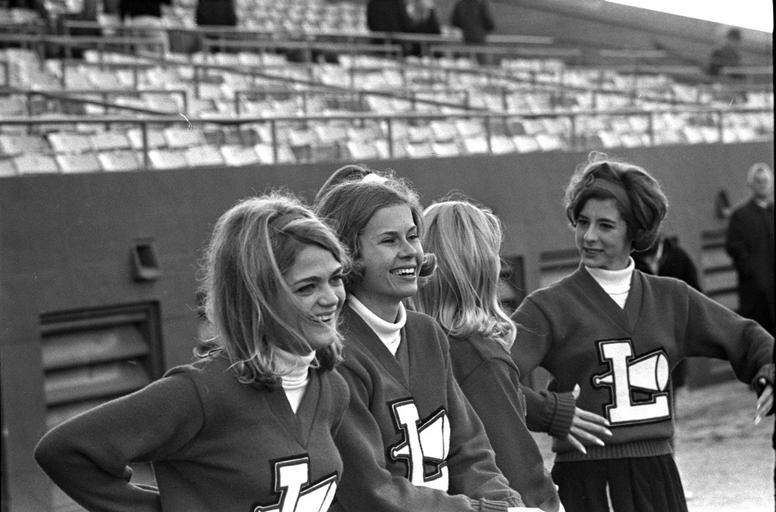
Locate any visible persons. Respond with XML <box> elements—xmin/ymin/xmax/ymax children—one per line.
<box><xmin>308</xmin><ymin>164</ymin><xmax>371</xmax><ymax>213</ymax></box>
<box><xmin>312</xmin><ymin>169</ymin><xmax>542</xmax><ymax>511</ymax></box>
<box><xmin>368</xmin><ymin>0</ymin><xmax>497</xmax><ymax>66</ymax></box>
<box><xmin>701</xmin><ymin>25</ymin><xmax>749</xmax><ymax>102</ymax></box>
<box><xmin>640</xmin><ymin>236</ymin><xmax>706</xmax><ymax>392</ymax></box>
<box><xmin>510</xmin><ymin>151</ymin><xmax>776</xmax><ymax>512</ymax></box>
<box><xmin>724</xmin><ymin>161</ymin><xmax>775</xmax><ymax>338</ymax></box>
<box><xmin>713</xmin><ymin>187</ymin><xmax>732</xmax><ymax>220</ymax></box>
<box><xmin>33</xmin><ymin>193</ymin><xmax>351</xmax><ymax>512</ymax></box>
<box><xmin>402</xmin><ymin>199</ymin><xmax>564</xmax><ymax>512</ymax></box>
<box><xmin>0</xmin><ymin>0</ymin><xmax>238</xmax><ymax>60</ymax></box>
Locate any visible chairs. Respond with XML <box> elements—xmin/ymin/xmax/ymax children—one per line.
<box><xmin>0</xmin><ymin>0</ymin><xmax>775</xmax><ymax>181</ymax></box>
